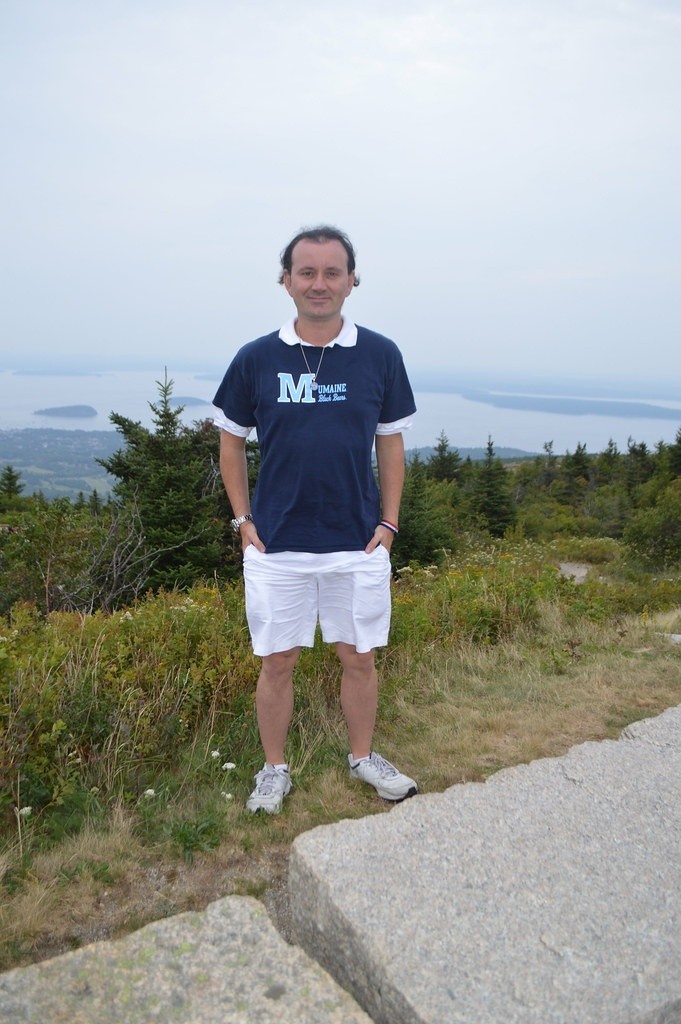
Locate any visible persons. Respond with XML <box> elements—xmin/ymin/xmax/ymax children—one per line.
<box><xmin>212</xmin><ymin>228</ymin><xmax>417</xmax><ymax>815</ymax></box>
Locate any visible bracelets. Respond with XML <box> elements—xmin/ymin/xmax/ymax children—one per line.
<box><xmin>380</xmin><ymin>520</ymin><xmax>398</xmax><ymax>534</ymax></box>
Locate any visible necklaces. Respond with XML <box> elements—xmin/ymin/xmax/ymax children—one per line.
<box><xmin>298</xmin><ymin>332</ymin><xmax>326</xmax><ymax>391</ymax></box>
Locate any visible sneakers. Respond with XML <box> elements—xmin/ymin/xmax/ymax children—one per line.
<box><xmin>348</xmin><ymin>752</ymin><xmax>418</xmax><ymax>801</ymax></box>
<box><xmin>246</xmin><ymin>762</ymin><xmax>293</xmax><ymax>816</ymax></box>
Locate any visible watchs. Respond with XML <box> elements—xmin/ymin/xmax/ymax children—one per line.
<box><xmin>231</xmin><ymin>514</ymin><xmax>252</xmax><ymax>533</ymax></box>
